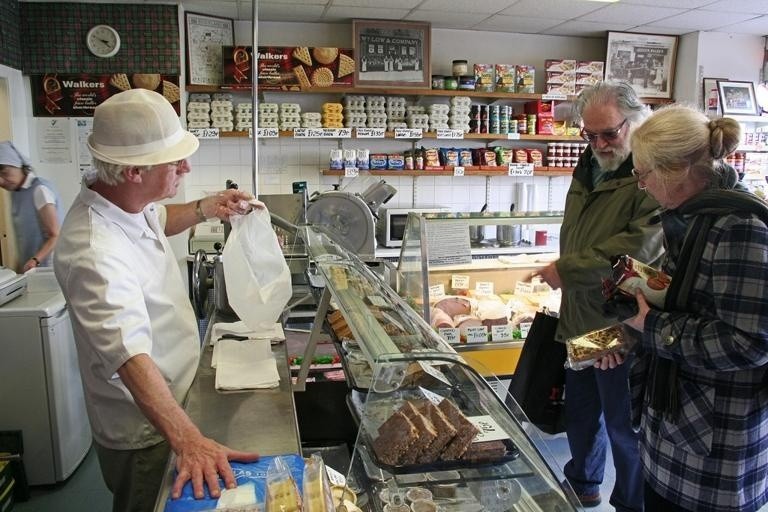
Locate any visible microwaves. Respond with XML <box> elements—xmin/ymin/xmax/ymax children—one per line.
<box><xmin>374</xmin><ymin>206</ymin><xmax>461</xmax><ymax>249</ymax></box>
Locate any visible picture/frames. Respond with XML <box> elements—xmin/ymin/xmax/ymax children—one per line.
<box><xmin>702</xmin><ymin>77</ymin><xmax>728</xmax><ymax>118</ymax></box>
<box><xmin>352</xmin><ymin>17</ymin><xmax>432</xmax><ymax>91</ymax></box>
<box><xmin>182</xmin><ymin>10</ymin><xmax>236</xmax><ymax>88</ymax></box>
<box><xmin>185</xmin><ymin>81</ymin><xmax>674</xmax><ymax>179</ymax></box>
<box><xmin>715</xmin><ymin>79</ymin><xmax>757</xmax><ymax>116</ymax></box>
<box><xmin>603</xmin><ymin>31</ymin><xmax>679</xmax><ymax>100</ymax></box>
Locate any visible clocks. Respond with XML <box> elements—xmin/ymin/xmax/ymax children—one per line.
<box><xmin>87</xmin><ymin>25</ymin><xmax>122</xmax><ymax>59</ymax></box>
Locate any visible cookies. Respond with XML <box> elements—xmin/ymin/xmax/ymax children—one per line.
<box><xmin>133</xmin><ymin>74</ymin><xmax>160</xmax><ymax>91</ymax></box>
<box><xmin>294</xmin><ymin>65</ymin><xmax>311</xmax><ymax>88</ymax></box>
<box><xmin>292</xmin><ymin>47</ymin><xmax>312</xmax><ymax>66</ymax></box>
<box><xmin>314</xmin><ymin>48</ymin><xmax>338</xmax><ymax>64</ymax></box>
<box><xmin>338</xmin><ymin>54</ymin><xmax>355</xmax><ymax>78</ymax></box>
<box><xmin>313</xmin><ymin>68</ymin><xmax>333</xmax><ymax>88</ymax></box>
<box><xmin>109</xmin><ymin>73</ymin><xmax>131</xmax><ymax>91</ymax></box>
<box><xmin>163</xmin><ymin>81</ymin><xmax>180</xmax><ymax>103</ymax></box>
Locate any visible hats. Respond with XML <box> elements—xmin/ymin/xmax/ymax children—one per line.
<box><xmin>87</xmin><ymin>88</ymin><xmax>199</xmax><ymax>165</ymax></box>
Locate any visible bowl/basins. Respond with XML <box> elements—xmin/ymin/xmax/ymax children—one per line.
<box><xmin>378</xmin><ymin>487</ymin><xmax>438</xmax><ymax>512</ymax></box>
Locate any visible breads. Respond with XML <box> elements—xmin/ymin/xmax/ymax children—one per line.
<box><xmin>329</xmin><ymin>308</ymin><xmax>352</xmax><ymax>339</ymax></box>
<box><xmin>373</xmin><ymin>398</ymin><xmax>507</xmax><ymax>465</ymax></box>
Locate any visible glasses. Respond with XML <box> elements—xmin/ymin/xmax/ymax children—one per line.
<box><xmin>580</xmin><ymin>119</ymin><xmax>626</xmax><ymax>141</ymax></box>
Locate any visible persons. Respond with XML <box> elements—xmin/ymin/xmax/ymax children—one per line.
<box><xmin>590</xmin><ymin>102</ymin><xmax>768</xmax><ymax>511</ymax></box>
<box><xmin>523</xmin><ymin>80</ymin><xmax>666</xmax><ymax>512</ymax></box>
<box><xmin>52</xmin><ymin>88</ymin><xmax>268</xmax><ymax>510</ymax></box>
<box><xmin>0</xmin><ymin>139</ymin><xmax>69</xmax><ymax>275</ymax></box>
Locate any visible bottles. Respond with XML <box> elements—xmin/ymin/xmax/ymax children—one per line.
<box><xmin>733</xmin><ymin>149</ymin><xmax>747</xmax><ymax>173</ymax></box>
<box><xmin>725</xmin><ymin>152</ymin><xmax>735</xmax><ymax>170</ymax></box>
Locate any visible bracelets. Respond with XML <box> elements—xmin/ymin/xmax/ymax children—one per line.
<box><xmin>29</xmin><ymin>257</ymin><xmax>41</xmax><ymax>265</ymax></box>
<box><xmin>194</xmin><ymin>199</ymin><xmax>208</xmax><ymax>223</ymax></box>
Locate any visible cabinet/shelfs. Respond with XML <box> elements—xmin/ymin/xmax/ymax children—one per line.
<box><xmin>720</xmin><ymin>115</ymin><xmax>767</xmax><ymax>207</ymax></box>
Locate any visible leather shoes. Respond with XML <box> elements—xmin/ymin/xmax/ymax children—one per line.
<box><xmin>533</xmin><ymin>486</ymin><xmax>602</xmax><ymax>507</ymax></box>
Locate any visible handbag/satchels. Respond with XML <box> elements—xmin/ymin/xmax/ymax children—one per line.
<box><xmin>505</xmin><ymin>312</ymin><xmax>567</xmax><ymax>434</ymax></box>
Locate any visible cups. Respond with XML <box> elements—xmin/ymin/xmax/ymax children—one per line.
<box><xmin>534</xmin><ymin>231</ymin><xmax>549</xmax><ymax>248</ymax></box>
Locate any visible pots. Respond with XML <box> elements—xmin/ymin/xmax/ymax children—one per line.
<box><xmin>497</xmin><ymin>212</ymin><xmax>521</xmax><ymax>246</ymax></box>
<box><xmin>456</xmin><ymin>209</ymin><xmax>488</xmax><ymax>245</ymax></box>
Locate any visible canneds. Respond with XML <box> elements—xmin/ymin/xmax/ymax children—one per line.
<box><xmin>445</xmin><ymin>76</ymin><xmax>458</xmax><ymax>90</ymax></box>
<box><xmin>432</xmin><ymin>75</ymin><xmax>445</xmax><ymax>90</ymax></box>
<box><xmin>370</xmin><ymin>155</ymin><xmax>405</xmax><ymax>170</ymax></box>
<box><xmin>452</xmin><ymin>60</ymin><xmax>468</xmax><ymax>76</ymax></box>
<box><xmin>460</xmin><ymin>76</ymin><xmax>475</xmax><ymax>90</ymax></box>
<box><xmin>470</xmin><ymin>105</ymin><xmax>519</xmax><ymax>134</ymax></box>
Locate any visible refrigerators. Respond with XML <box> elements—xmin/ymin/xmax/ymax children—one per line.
<box><xmin>0</xmin><ymin>290</ymin><xmax>96</xmax><ymax>488</ymax></box>
